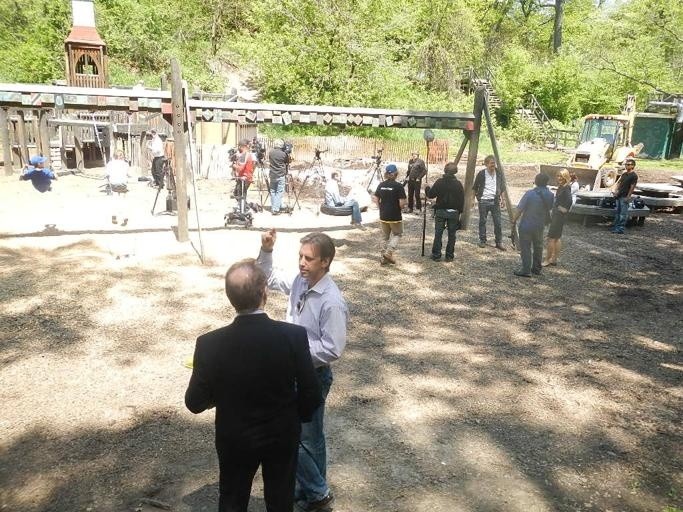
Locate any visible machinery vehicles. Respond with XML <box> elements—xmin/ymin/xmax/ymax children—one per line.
<box><xmin>538</xmin><ymin>114</ymin><xmax>644</xmax><ymax>192</ymax></box>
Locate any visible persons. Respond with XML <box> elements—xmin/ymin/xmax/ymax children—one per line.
<box><xmin>323</xmin><ymin>170</ymin><xmax>368</xmax><ymax>231</ymax></box>
<box><xmin>147</xmin><ymin>128</ymin><xmax>165</xmax><ymax>186</ymax></box>
<box><xmin>104</xmin><ymin>149</ymin><xmax>134</xmax><ymax>226</ymax></box>
<box><xmin>269</xmin><ymin>139</ymin><xmax>292</xmax><ymax>215</ymax></box>
<box><xmin>18</xmin><ymin>156</ymin><xmax>71</xmax><ymax>193</ymax></box>
<box><xmin>608</xmin><ymin>159</ymin><xmax>639</xmax><ymax>234</ymax></box>
<box><xmin>231</xmin><ymin>139</ymin><xmax>254</xmax><ymax>214</ymax></box>
<box><xmin>255</xmin><ymin>228</ymin><xmax>349</xmax><ymax>512</ymax></box>
<box><xmin>373</xmin><ymin>150</ymin><xmax>579</xmax><ymax>277</ymax></box>
<box><xmin>185</xmin><ymin>257</ymin><xmax>323</xmax><ymax>512</ymax></box>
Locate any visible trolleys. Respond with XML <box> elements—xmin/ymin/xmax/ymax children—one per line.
<box><xmin>223</xmin><ymin>176</ymin><xmax>256</xmax><ymax>228</ymax></box>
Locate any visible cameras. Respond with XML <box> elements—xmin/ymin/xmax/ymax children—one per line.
<box><xmin>316</xmin><ymin>147</ymin><xmax>328</xmax><ymax>155</ymax></box>
<box><xmin>371</xmin><ymin>148</ymin><xmax>384</xmax><ymax>161</ymax></box>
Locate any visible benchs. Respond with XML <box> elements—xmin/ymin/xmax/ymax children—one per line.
<box><xmin>552</xmin><ymin>173</ymin><xmax>683</xmax><ymax>228</ymax></box>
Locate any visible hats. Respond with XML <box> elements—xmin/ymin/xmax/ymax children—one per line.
<box><xmin>385</xmin><ymin>165</ymin><xmax>398</xmax><ymax>174</ymax></box>
<box><xmin>31</xmin><ymin>155</ymin><xmax>49</xmax><ymax>165</ymax></box>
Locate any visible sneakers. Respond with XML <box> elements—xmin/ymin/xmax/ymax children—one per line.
<box><xmin>430</xmin><ymin>253</ymin><xmax>454</xmax><ymax>262</ymax></box>
<box><xmin>514</xmin><ymin>260</ymin><xmax>557</xmax><ymax>277</ymax></box>
<box><xmin>478</xmin><ymin>240</ymin><xmax>506</xmax><ymax>251</ymax></box>
<box><xmin>381</xmin><ymin>255</ymin><xmax>396</xmax><ymax>265</ymax></box>
<box><xmin>608</xmin><ymin>226</ymin><xmax>626</xmax><ymax>233</ymax></box>
<box><xmin>403</xmin><ymin>207</ymin><xmax>422</xmax><ymax>215</ymax></box>
<box><xmin>351</xmin><ymin>222</ymin><xmax>367</xmax><ymax>231</ymax></box>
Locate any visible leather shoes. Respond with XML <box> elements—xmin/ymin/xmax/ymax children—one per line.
<box><xmin>294</xmin><ymin>486</ymin><xmax>335</xmax><ymax>512</ymax></box>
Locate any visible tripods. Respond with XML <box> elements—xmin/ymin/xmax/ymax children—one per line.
<box><xmin>366</xmin><ymin>161</ymin><xmax>384</xmax><ymax>191</ymax></box>
<box><xmin>151</xmin><ymin>158</ymin><xmax>176</xmax><ymax>215</ymax></box>
<box><xmin>290</xmin><ymin>155</ymin><xmax>327</xmax><ymax>216</ymax></box>
<box><xmin>249</xmin><ymin>156</ymin><xmax>301</xmax><ymax>211</ymax></box>
